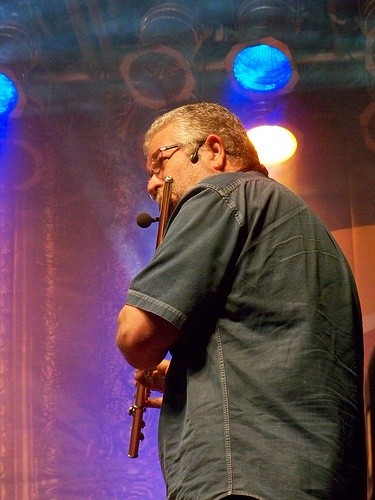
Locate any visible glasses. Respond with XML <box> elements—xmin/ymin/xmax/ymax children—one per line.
<box><xmin>146</xmin><ymin>139</ymin><xmax>207</xmax><ymax>180</ymax></box>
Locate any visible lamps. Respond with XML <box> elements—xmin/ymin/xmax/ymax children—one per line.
<box><xmin>0</xmin><ymin>13</ymin><xmax>41</xmax><ymax>115</ymax></box>
<box><xmin>224</xmin><ymin>1</ymin><xmax>301</xmax><ymax>99</ymax></box>
<box><xmin>330</xmin><ymin>1</ymin><xmax>375</xmax><ymax>78</ymax></box>
<box><xmin>0</xmin><ymin>93</ymin><xmax>48</xmax><ymax>190</ymax></box>
<box><xmin>243</xmin><ymin>98</ymin><xmax>299</xmax><ymax>166</ymax></box>
<box><xmin>121</xmin><ymin>3</ymin><xmax>214</xmax><ymax>111</ymax></box>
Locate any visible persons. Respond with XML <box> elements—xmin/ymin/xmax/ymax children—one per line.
<box><xmin>113</xmin><ymin>102</ymin><xmax>367</xmax><ymax>500</ymax></box>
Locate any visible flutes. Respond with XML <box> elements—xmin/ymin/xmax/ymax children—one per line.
<box><xmin>126</xmin><ymin>176</ymin><xmax>175</xmax><ymax>458</ymax></box>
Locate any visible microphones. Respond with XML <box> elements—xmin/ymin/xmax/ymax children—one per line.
<box><xmin>136</xmin><ymin>213</ymin><xmax>160</xmax><ymax>229</ymax></box>
